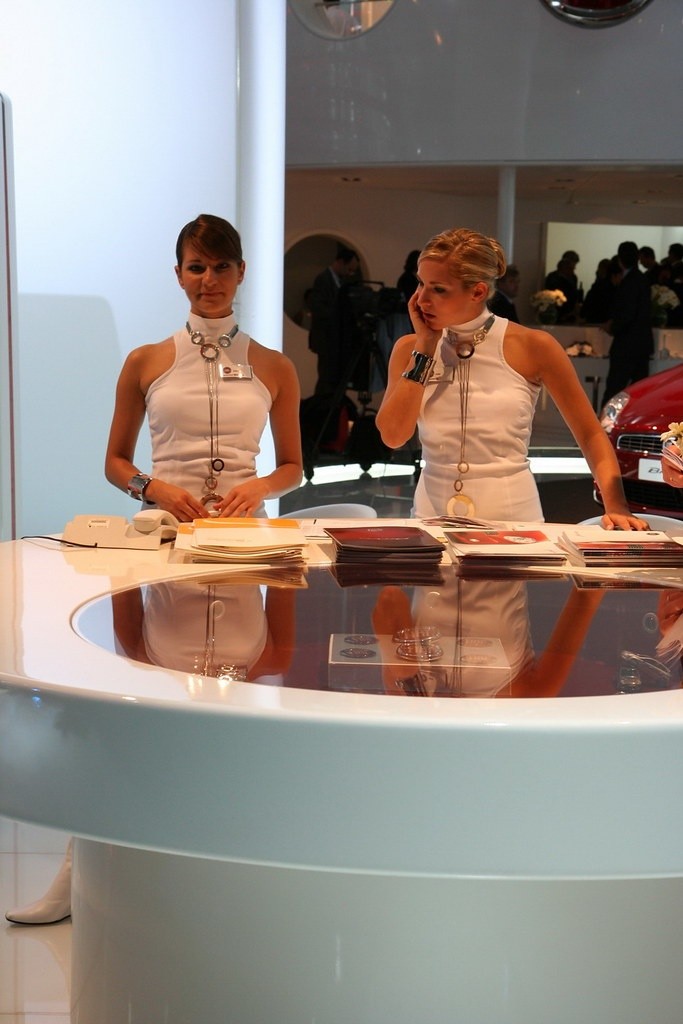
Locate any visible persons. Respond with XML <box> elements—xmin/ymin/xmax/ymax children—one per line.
<box><xmin>308</xmin><ymin>242</ymin><xmax>683</xmax><ymax>459</ymax></box>
<box><xmin>661</xmin><ymin>446</ymin><xmax>683</xmax><ymax>488</ymax></box>
<box><xmin>370</xmin><ymin>569</ymin><xmax>607</xmax><ymax>698</ymax></box>
<box><xmin>112</xmin><ymin>580</ymin><xmax>296</xmax><ymax>684</ymax></box>
<box><xmin>5</xmin><ymin>215</ymin><xmax>304</xmax><ymax>925</ymax></box>
<box><xmin>374</xmin><ymin>229</ymin><xmax>650</xmax><ymax>534</ymax></box>
<box><xmin>657</xmin><ymin>591</ymin><xmax>683</xmax><ymax>637</ymax></box>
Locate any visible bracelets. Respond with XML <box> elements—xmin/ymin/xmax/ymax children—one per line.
<box><xmin>402</xmin><ymin>350</ymin><xmax>436</xmax><ymax>387</ymax></box>
<box><xmin>127</xmin><ymin>474</ymin><xmax>156</xmax><ymax>505</ymax></box>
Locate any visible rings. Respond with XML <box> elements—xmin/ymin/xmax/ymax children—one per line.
<box><xmin>666</xmin><ymin>596</ymin><xmax>669</xmax><ymax>601</ymax></box>
<box><xmin>670</xmin><ymin>477</ymin><xmax>673</xmax><ymax>482</ymax></box>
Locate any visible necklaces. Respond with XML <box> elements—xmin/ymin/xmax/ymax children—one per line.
<box><xmin>447</xmin><ymin>581</ymin><xmax>466</xmax><ymax>694</ymax></box>
<box><xmin>186</xmin><ymin>322</ymin><xmax>239</xmax><ymax>516</ymax></box>
<box><xmin>194</xmin><ymin>584</ymin><xmax>219</xmax><ymax>677</ymax></box>
<box><xmin>442</xmin><ymin>314</ymin><xmax>494</xmax><ymax>516</ymax></box>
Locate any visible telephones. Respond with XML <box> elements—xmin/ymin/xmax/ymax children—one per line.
<box><xmin>61</xmin><ymin>508</ymin><xmax>180</xmax><ymax>551</ymax></box>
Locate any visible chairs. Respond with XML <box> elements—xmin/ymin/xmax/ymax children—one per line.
<box><xmin>577</xmin><ymin>512</ymin><xmax>683</xmax><ymax>532</ymax></box>
<box><xmin>276</xmin><ymin>503</ymin><xmax>378</xmax><ymax>520</ymax></box>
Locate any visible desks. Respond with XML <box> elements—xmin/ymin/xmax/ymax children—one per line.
<box><xmin>0</xmin><ymin>517</ymin><xmax>683</xmax><ymax>1024</ymax></box>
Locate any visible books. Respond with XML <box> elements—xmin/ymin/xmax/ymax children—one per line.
<box><xmin>456</xmin><ymin>566</ymin><xmax>566</xmax><ymax>581</ymax></box>
<box><xmin>183</xmin><ymin>567</ymin><xmax>308</xmax><ymax>588</ymax></box>
<box><xmin>174</xmin><ymin>518</ymin><xmax>683</xmax><ymax>567</ymax></box>
<box><xmin>329</xmin><ymin>564</ymin><xmax>446</xmax><ymax>587</ymax></box>
<box><xmin>571</xmin><ymin>570</ymin><xmax>682</xmax><ymax>590</ymax></box>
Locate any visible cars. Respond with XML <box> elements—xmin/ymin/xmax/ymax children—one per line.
<box><xmin>591</xmin><ymin>363</ymin><xmax>682</xmax><ymax>520</ymax></box>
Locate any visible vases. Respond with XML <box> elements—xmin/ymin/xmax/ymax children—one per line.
<box><xmin>537</xmin><ymin>305</ymin><xmax>560</xmax><ymax>324</ymax></box>
<box><xmin>651</xmin><ymin>306</ymin><xmax>668</xmax><ymax>328</ymax></box>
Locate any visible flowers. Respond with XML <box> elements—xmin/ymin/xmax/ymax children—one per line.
<box><xmin>659</xmin><ymin>422</ymin><xmax>683</xmax><ymax>488</ymax></box>
<box><xmin>650</xmin><ymin>284</ymin><xmax>680</xmax><ymax>310</ymax></box>
<box><xmin>528</xmin><ymin>288</ymin><xmax>568</xmax><ymax>312</ymax></box>
